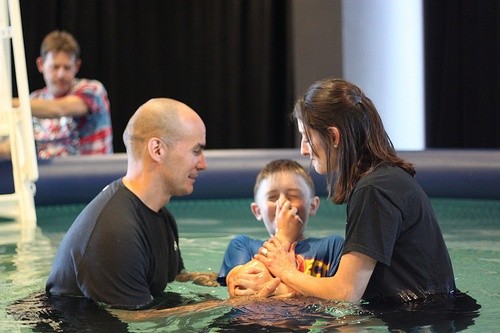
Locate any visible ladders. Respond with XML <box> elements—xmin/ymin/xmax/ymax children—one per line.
<box><xmin>0</xmin><ymin>0</ymin><xmax>39</xmax><ymax>233</ymax></box>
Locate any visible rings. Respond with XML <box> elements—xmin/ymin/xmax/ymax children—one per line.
<box><xmin>261</xmin><ymin>248</ymin><xmax>269</xmax><ymax>255</ymax></box>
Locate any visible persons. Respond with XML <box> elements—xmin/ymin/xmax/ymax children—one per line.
<box><xmin>0</xmin><ymin>31</ymin><xmax>113</xmax><ymax>160</ymax></box>
<box><xmin>45</xmin><ymin>98</ymin><xmax>294</xmax><ymax>322</ymax></box>
<box><xmin>217</xmin><ymin>159</ymin><xmax>346</xmax><ymax>297</ymax></box>
<box><xmin>254</xmin><ymin>78</ymin><xmax>456</xmax><ymax>305</ymax></box>
